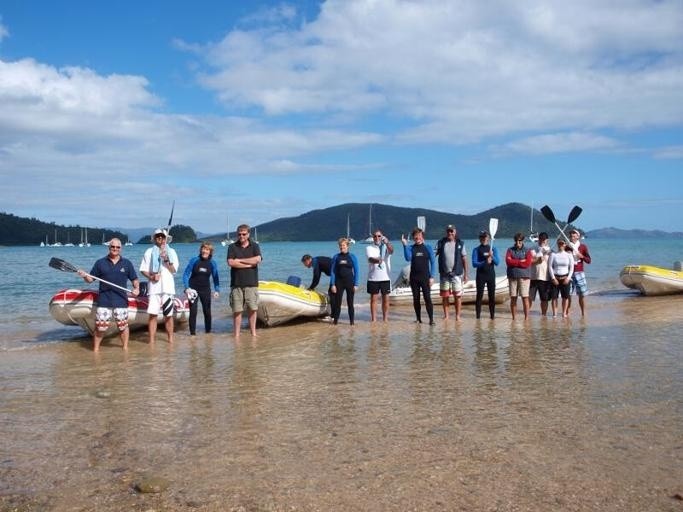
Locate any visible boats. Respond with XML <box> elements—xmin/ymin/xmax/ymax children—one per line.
<box><xmin>48</xmin><ymin>286</ymin><xmax>198</xmax><ymax>336</ymax></box>
<box><xmin>255</xmin><ymin>281</ymin><xmax>335</xmax><ymax>327</ymax></box>
<box><xmin>618</xmin><ymin>261</ymin><xmax>682</xmax><ymax>296</ymax></box>
<box><xmin>388</xmin><ymin>275</ymin><xmax>510</xmax><ymax>307</ymax></box>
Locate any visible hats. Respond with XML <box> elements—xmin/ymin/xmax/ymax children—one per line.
<box><xmin>150</xmin><ymin>227</ymin><xmax>172</xmax><ymax>244</ymax></box>
<box><xmin>568</xmin><ymin>229</ymin><xmax>579</xmax><ymax>236</ymax></box>
<box><xmin>445</xmin><ymin>224</ymin><xmax>455</xmax><ymax>229</ymax></box>
<box><xmin>478</xmin><ymin>229</ymin><xmax>488</xmax><ymax>237</ymax></box>
<box><xmin>557</xmin><ymin>240</ymin><xmax>564</xmax><ymax>244</ymax></box>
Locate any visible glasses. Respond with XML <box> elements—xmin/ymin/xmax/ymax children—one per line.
<box><xmin>156</xmin><ymin>234</ymin><xmax>164</xmax><ymax>238</ymax></box>
<box><xmin>372</xmin><ymin>235</ymin><xmax>381</xmax><ymax>238</ymax></box>
<box><xmin>237</xmin><ymin>233</ymin><xmax>247</xmax><ymax>236</ymax></box>
<box><xmin>110</xmin><ymin>246</ymin><xmax>120</xmax><ymax>249</ymax></box>
<box><xmin>569</xmin><ymin>231</ymin><xmax>575</xmax><ymax>234</ymax></box>
<box><xmin>514</xmin><ymin>239</ymin><xmax>521</xmax><ymax>242</ymax></box>
<box><xmin>446</xmin><ymin>229</ymin><xmax>453</xmax><ymax>233</ymax></box>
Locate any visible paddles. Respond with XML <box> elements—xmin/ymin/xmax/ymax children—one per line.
<box><xmin>543</xmin><ymin>206</ymin><xmax>582</xmax><ymax>254</ymax></box>
<box><xmin>489</xmin><ymin>218</ymin><xmax>497</xmax><ymax>250</ymax></box>
<box><xmin>417</xmin><ymin>216</ymin><xmax>425</xmax><ymax>233</ymax></box>
<box><xmin>540</xmin><ymin>205</ymin><xmax>578</xmax><ymax>253</ymax></box>
<box><xmin>157</xmin><ymin>200</ymin><xmax>175</xmax><ymax>277</ymax></box>
<box><xmin>48</xmin><ymin>257</ymin><xmax>132</xmax><ymax>294</ymax></box>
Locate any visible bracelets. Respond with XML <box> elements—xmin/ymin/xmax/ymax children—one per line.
<box><xmin>164</xmin><ymin>257</ymin><xmax>169</xmax><ymax>262</ymax></box>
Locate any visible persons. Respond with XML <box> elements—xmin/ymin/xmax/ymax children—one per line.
<box><xmin>228</xmin><ymin>225</ymin><xmax>263</xmax><ymax>337</ymax></box>
<box><xmin>565</xmin><ymin>229</ymin><xmax>592</xmax><ymax>316</ymax></box>
<box><xmin>505</xmin><ymin>232</ymin><xmax>532</xmax><ymax>320</ymax></box>
<box><xmin>139</xmin><ymin>229</ymin><xmax>179</xmax><ymax>344</ymax></box>
<box><xmin>78</xmin><ymin>237</ymin><xmax>140</xmax><ymax>351</ymax></box>
<box><xmin>366</xmin><ymin>230</ymin><xmax>394</xmax><ymax>323</ymax></box>
<box><xmin>433</xmin><ymin>224</ymin><xmax>468</xmax><ymax>321</ymax></box>
<box><xmin>301</xmin><ymin>255</ymin><xmax>335</xmax><ymax>318</ymax></box>
<box><xmin>401</xmin><ymin>228</ymin><xmax>436</xmax><ymax>325</ymax></box>
<box><xmin>548</xmin><ymin>238</ymin><xmax>575</xmax><ymax>317</ymax></box>
<box><xmin>472</xmin><ymin>230</ymin><xmax>499</xmax><ymax>320</ymax></box>
<box><xmin>330</xmin><ymin>237</ymin><xmax>360</xmax><ymax>325</ymax></box>
<box><xmin>529</xmin><ymin>232</ymin><xmax>551</xmax><ymax>316</ymax></box>
<box><xmin>183</xmin><ymin>242</ymin><xmax>220</xmax><ymax>335</ymax></box>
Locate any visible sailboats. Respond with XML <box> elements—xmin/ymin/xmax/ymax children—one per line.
<box><xmin>338</xmin><ymin>199</ymin><xmax>385</xmax><ymax>244</ymax></box>
<box><xmin>39</xmin><ymin>227</ymin><xmax>133</xmax><ymax>248</ymax></box>
<box><xmin>220</xmin><ymin>215</ymin><xmax>260</xmax><ymax>247</ymax></box>
